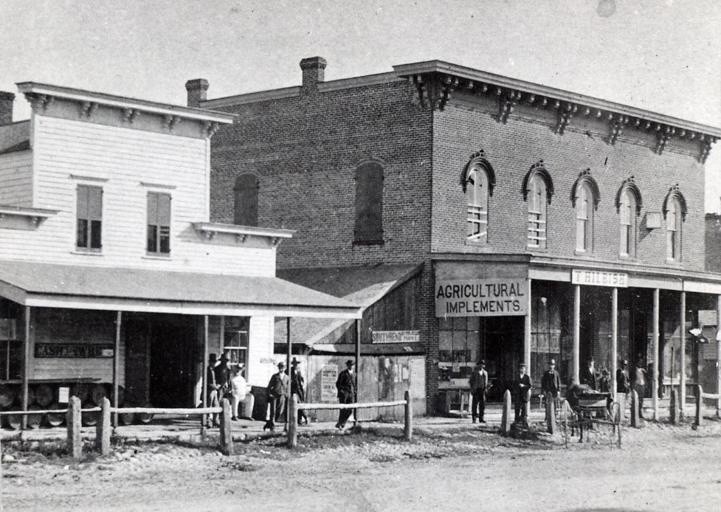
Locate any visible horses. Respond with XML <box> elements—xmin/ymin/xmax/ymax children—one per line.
<box><xmin>565</xmin><ymin>375</ymin><xmax>594</xmax><ymax>431</ymax></box>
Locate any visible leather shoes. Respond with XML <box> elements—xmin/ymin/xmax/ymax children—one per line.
<box><xmin>472</xmin><ymin>419</ymin><xmax>486</xmax><ymax>424</ymax></box>
<box><xmin>207</xmin><ymin>416</ymin><xmax>255</xmax><ymax>428</ymax></box>
<box><xmin>336</xmin><ymin>423</ymin><xmax>344</xmax><ymax>428</ymax></box>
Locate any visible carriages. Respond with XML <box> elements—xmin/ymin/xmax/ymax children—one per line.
<box><xmin>561</xmin><ymin>375</ymin><xmax>622</xmax><ymax>448</ymax></box>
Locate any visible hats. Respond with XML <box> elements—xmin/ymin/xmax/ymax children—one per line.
<box><xmin>622</xmin><ymin>359</ymin><xmax>628</xmax><ymax>365</ymax></box>
<box><xmin>208</xmin><ymin>354</ymin><xmax>246</xmax><ymax>370</ymax></box>
<box><xmin>586</xmin><ymin>356</ymin><xmax>595</xmax><ymax>361</ymax></box>
<box><xmin>477</xmin><ymin>360</ymin><xmax>487</xmax><ymax>365</ymax></box>
<box><xmin>548</xmin><ymin>358</ymin><xmax>556</xmax><ymax>365</ymax></box>
<box><xmin>345</xmin><ymin>360</ymin><xmax>354</xmax><ymax>365</ymax></box>
<box><xmin>519</xmin><ymin>361</ymin><xmax>527</xmax><ymax>366</ymax></box>
<box><xmin>277</xmin><ymin>357</ymin><xmax>300</xmax><ymax>367</ymax></box>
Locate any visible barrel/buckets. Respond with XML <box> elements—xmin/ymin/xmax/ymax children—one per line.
<box><xmin>0</xmin><ymin>382</ymin><xmax>155</xmax><ymax>430</ymax></box>
<box><xmin>239</xmin><ymin>393</ymin><xmax>255</xmax><ymax>417</ymax></box>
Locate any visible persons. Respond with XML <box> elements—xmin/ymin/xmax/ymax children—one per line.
<box><xmin>468</xmin><ymin>359</ymin><xmax>489</xmax><ymax>423</ymax></box>
<box><xmin>541</xmin><ymin>364</ymin><xmax>562</xmax><ymax>420</ymax></box>
<box><xmin>513</xmin><ymin>364</ymin><xmax>532</xmax><ymax>420</ymax></box>
<box><xmin>284</xmin><ymin>362</ymin><xmax>309</xmax><ymax>425</ymax></box>
<box><xmin>583</xmin><ymin>355</ymin><xmax>649</xmax><ymax>420</ymax></box>
<box><xmin>267</xmin><ymin>361</ymin><xmax>291</xmax><ymax>425</ymax></box>
<box><xmin>229</xmin><ymin>368</ymin><xmax>256</xmax><ymax>421</ymax></box>
<box><xmin>336</xmin><ymin>359</ymin><xmax>357</xmax><ymax>428</ymax></box>
<box><xmin>200</xmin><ymin>360</ymin><xmax>222</xmax><ymax>427</ymax></box>
<box><xmin>214</xmin><ymin>360</ymin><xmax>232</xmax><ymax>407</ymax></box>
